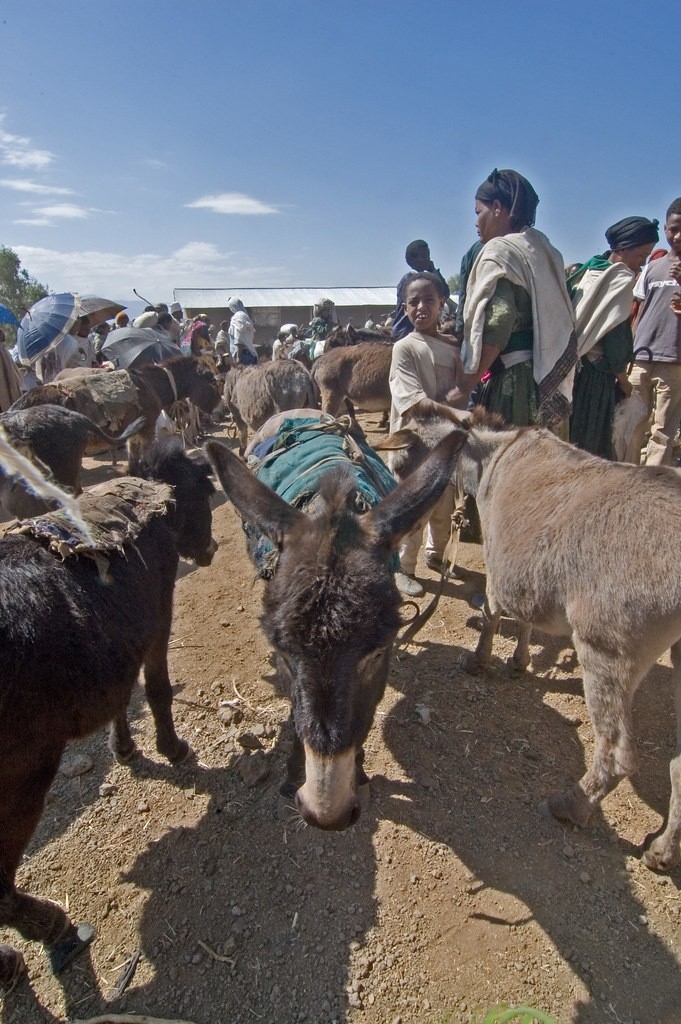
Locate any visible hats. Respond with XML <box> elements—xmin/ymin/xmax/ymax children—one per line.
<box><xmin>169</xmin><ymin>303</ymin><xmax>182</xmax><ymax>314</ymax></box>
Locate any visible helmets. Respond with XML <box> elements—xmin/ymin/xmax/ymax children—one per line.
<box><xmin>116</xmin><ymin>314</ymin><xmax>131</xmax><ymax>325</ymax></box>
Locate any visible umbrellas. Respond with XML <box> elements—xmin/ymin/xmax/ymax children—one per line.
<box><xmin>78</xmin><ymin>296</ymin><xmax>128</xmax><ymax>328</ymax></box>
<box><xmin>17</xmin><ymin>292</ymin><xmax>81</xmax><ymax>366</ymax></box>
<box><xmin>0</xmin><ymin>303</ymin><xmax>24</xmax><ymax>331</ymax></box>
<box><xmin>100</xmin><ymin>327</ymin><xmax>184</xmax><ymax>371</ymax></box>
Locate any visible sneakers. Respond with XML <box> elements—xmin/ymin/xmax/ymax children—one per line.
<box><xmin>426</xmin><ymin>556</ymin><xmax>462</xmax><ymax>579</ymax></box>
<box><xmin>394</xmin><ymin>570</ymin><xmax>424</xmax><ymax>595</ymax></box>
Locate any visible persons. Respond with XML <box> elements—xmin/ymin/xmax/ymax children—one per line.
<box><xmin>388</xmin><ymin>272</ymin><xmax>473</xmax><ymax>595</ymax></box>
<box><xmin>12</xmin><ymin>296</ymin><xmax>258</xmax><ymax>395</ymax></box>
<box><xmin>0</xmin><ymin>329</ymin><xmax>23</xmax><ymax>413</ymax></box>
<box><xmin>393</xmin><ymin>169</ymin><xmax>681</xmax><ymax>545</ymax></box>
<box><xmin>272</xmin><ymin>297</ymin><xmax>388</xmax><ymax>362</ymax></box>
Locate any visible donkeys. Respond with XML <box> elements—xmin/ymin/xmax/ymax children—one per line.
<box><xmin>0</xmin><ymin>324</ymin><xmax>681</xmax><ymax>986</ymax></box>
